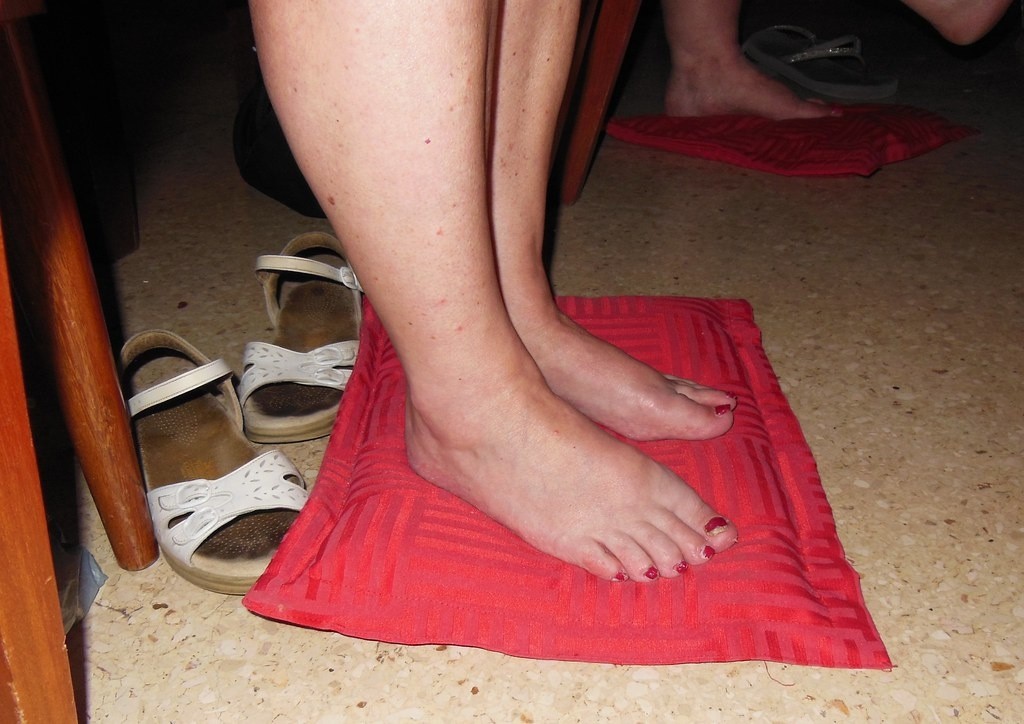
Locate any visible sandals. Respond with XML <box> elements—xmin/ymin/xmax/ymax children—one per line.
<box><xmin>238</xmin><ymin>231</ymin><xmax>364</xmax><ymax>444</ymax></box>
<box><xmin>119</xmin><ymin>329</ymin><xmax>310</xmax><ymax>595</ymax></box>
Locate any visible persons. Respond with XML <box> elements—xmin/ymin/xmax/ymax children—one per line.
<box><xmin>662</xmin><ymin>0</ymin><xmax>1012</xmax><ymax>123</ymax></box>
<box><xmin>246</xmin><ymin>0</ymin><xmax>739</xmax><ymax>584</ymax></box>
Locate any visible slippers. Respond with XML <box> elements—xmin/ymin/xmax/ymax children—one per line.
<box><xmin>746</xmin><ymin>25</ymin><xmax>898</xmax><ymax>100</ymax></box>
